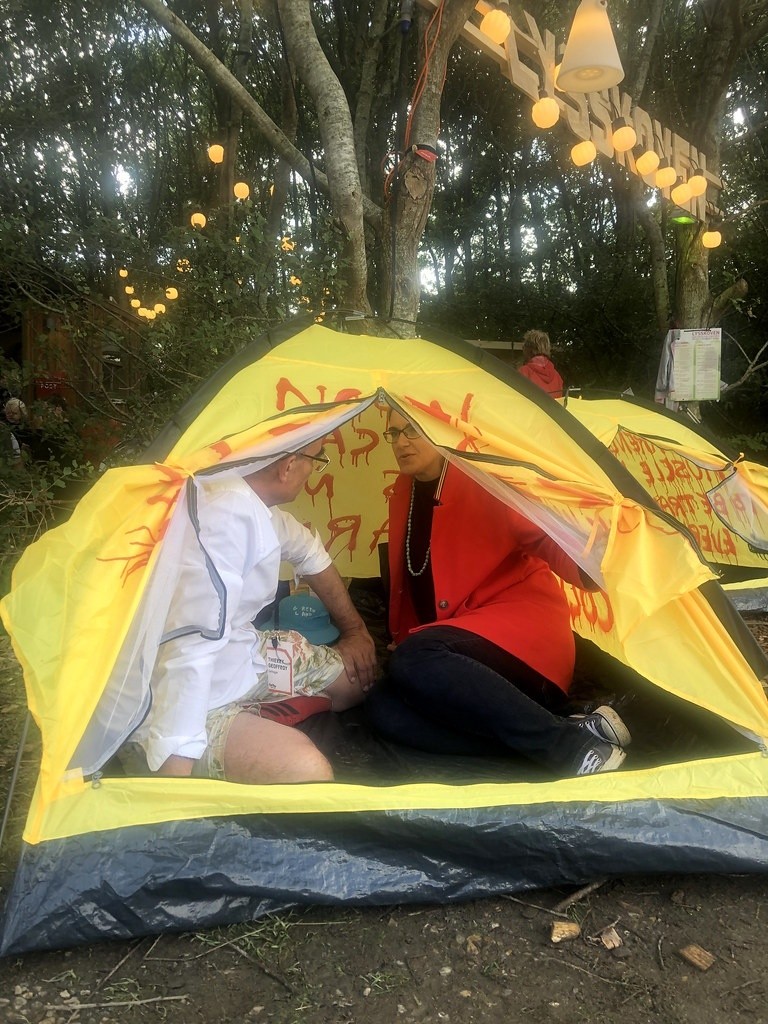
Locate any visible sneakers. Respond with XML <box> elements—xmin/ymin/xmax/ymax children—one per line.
<box><xmin>238</xmin><ymin>692</ymin><xmax>333</xmax><ymax>727</ymax></box>
<box><xmin>568</xmin><ymin>705</ymin><xmax>631</xmax><ymax>777</ymax></box>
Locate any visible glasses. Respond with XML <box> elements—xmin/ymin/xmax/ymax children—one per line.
<box><xmin>297</xmin><ymin>450</ymin><xmax>330</xmax><ymax>473</ymax></box>
<box><xmin>383</xmin><ymin>426</ymin><xmax>422</xmax><ymax>444</ymax></box>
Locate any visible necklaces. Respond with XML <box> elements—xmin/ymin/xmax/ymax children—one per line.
<box><xmin>407</xmin><ymin>478</ymin><xmax>439</xmax><ymax>576</ymax></box>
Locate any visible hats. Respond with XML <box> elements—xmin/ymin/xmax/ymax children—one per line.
<box><xmin>259</xmin><ymin>594</ymin><xmax>340</xmax><ymax>645</ymax></box>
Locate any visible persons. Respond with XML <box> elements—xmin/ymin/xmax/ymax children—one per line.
<box><xmin>365</xmin><ymin>406</ymin><xmax>633</xmax><ymax>776</ymax></box>
<box><xmin>517</xmin><ymin>330</ymin><xmax>563</xmax><ymax>398</ymax></box>
<box><xmin>0</xmin><ymin>423</ymin><xmax>29</xmax><ymax>472</ymax></box>
<box><xmin>98</xmin><ymin>438</ymin><xmax>379</xmax><ymax>784</ymax></box>
<box><xmin>33</xmin><ymin>395</ymin><xmax>83</xmax><ymax>468</ymax></box>
<box><xmin>4</xmin><ymin>398</ymin><xmax>29</xmax><ymax>430</ymax></box>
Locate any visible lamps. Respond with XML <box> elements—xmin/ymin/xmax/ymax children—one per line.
<box><xmin>115</xmin><ymin>0</ymin><xmax>725</xmax><ymax>323</ymax></box>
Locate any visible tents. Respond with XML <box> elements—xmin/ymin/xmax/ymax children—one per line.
<box><xmin>2</xmin><ymin>312</ymin><xmax>767</xmax><ymax>958</ymax></box>
<box><xmin>544</xmin><ymin>389</ymin><xmax>767</xmax><ymax>615</ymax></box>
